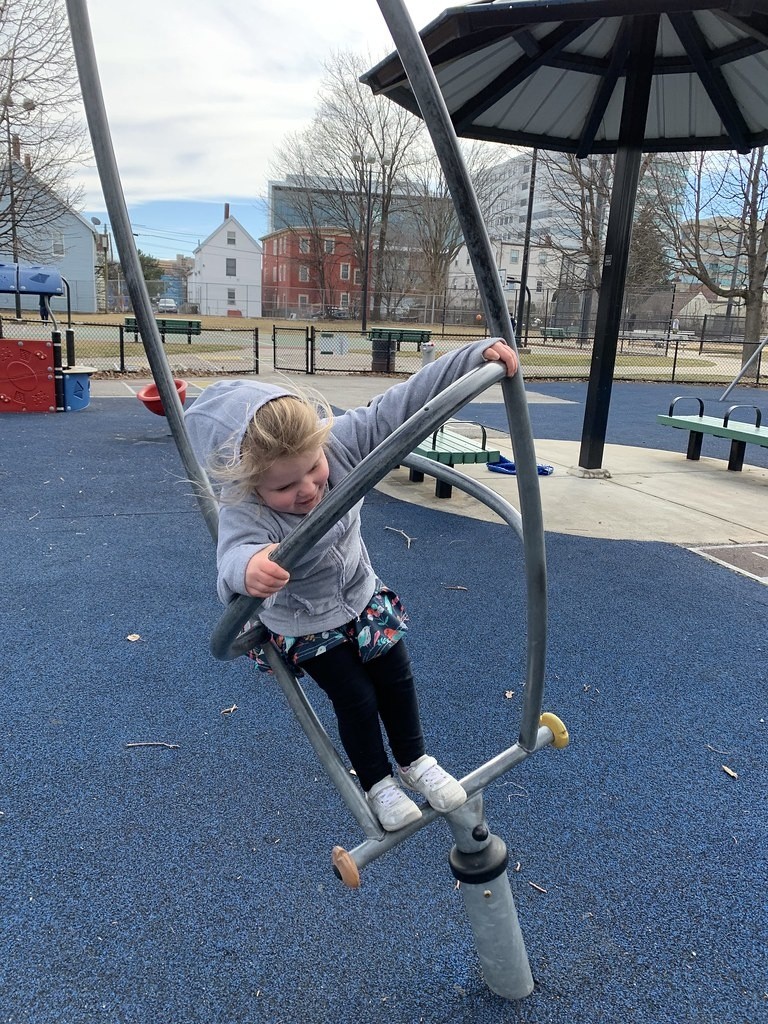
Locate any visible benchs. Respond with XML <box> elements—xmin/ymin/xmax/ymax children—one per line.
<box><xmin>393</xmin><ymin>421</ymin><xmax>500</xmax><ymax>499</ymax></box>
<box><xmin>657</xmin><ymin>396</ymin><xmax>768</xmax><ymax>472</ymax></box>
<box><xmin>124</xmin><ymin>318</ymin><xmax>202</xmax><ymax>345</ymax></box>
<box><xmin>540</xmin><ymin>327</ymin><xmax>565</xmax><ymax>342</ymax></box>
<box><xmin>366</xmin><ymin>327</ymin><xmax>432</xmax><ymax>352</ymax></box>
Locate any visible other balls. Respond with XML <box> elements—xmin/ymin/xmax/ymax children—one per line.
<box><xmin>476</xmin><ymin>315</ymin><xmax>481</xmax><ymax>320</ymax></box>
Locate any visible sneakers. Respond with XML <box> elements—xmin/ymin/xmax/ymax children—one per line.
<box><xmin>398</xmin><ymin>753</ymin><xmax>468</xmax><ymax>813</ymax></box>
<box><xmin>364</xmin><ymin>775</ymin><xmax>422</xmax><ymax>830</ymax></box>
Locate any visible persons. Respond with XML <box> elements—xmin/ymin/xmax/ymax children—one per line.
<box><xmin>510</xmin><ymin>312</ymin><xmax>517</xmax><ymax>332</ymax></box>
<box><xmin>185</xmin><ymin>335</ymin><xmax>520</xmax><ymax>831</ymax></box>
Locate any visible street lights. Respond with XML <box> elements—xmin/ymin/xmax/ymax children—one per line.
<box><xmin>506</xmin><ymin>279</ymin><xmax>531</xmax><ymax>347</ymax></box>
<box><xmin>665</xmin><ymin>260</ymin><xmax>685</xmax><ymax>357</ymax></box>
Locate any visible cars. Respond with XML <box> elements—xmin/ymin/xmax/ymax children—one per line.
<box><xmin>156</xmin><ymin>298</ymin><xmax>177</xmax><ymax>313</ymax></box>
